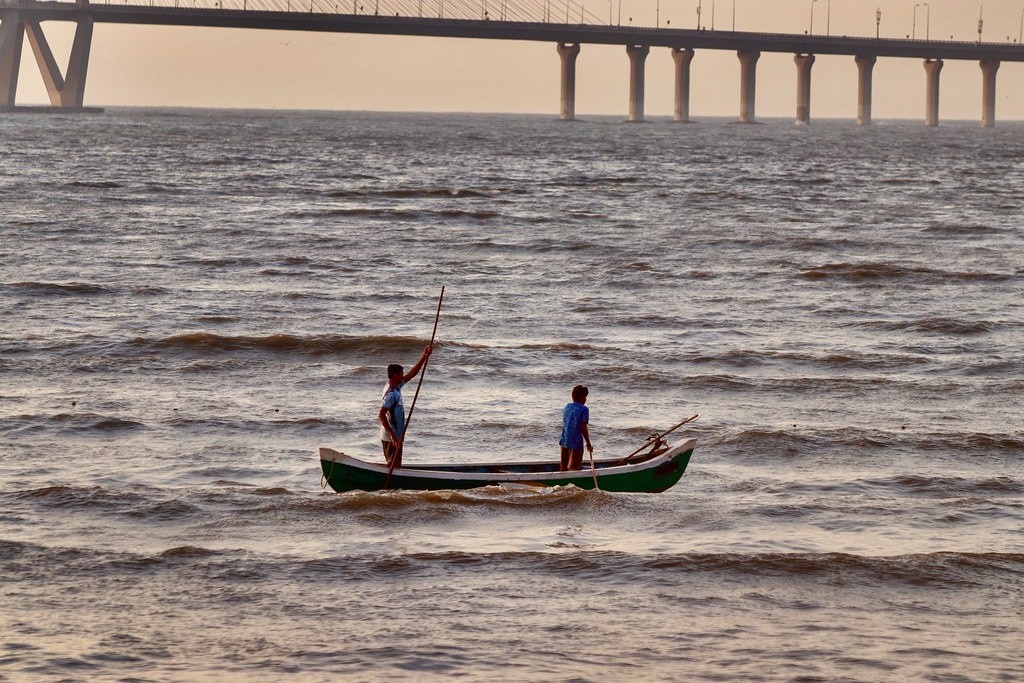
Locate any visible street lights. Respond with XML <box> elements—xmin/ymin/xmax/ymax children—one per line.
<box><xmin>912</xmin><ymin>3</ymin><xmax>920</xmax><ymax>40</ymax></box>
<box><xmin>809</xmin><ymin>0</ymin><xmax>818</xmax><ymax>35</ymax></box>
<box><xmin>923</xmin><ymin>3</ymin><xmax>929</xmax><ymax>41</ymax></box>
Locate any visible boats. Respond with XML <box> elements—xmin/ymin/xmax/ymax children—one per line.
<box><xmin>317</xmin><ymin>436</ymin><xmax>699</xmax><ymax>494</ymax></box>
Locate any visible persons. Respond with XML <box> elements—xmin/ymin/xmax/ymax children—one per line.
<box><xmin>378</xmin><ymin>345</ymin><xmax>433</xmax><ymax>469</ymax></box>
<box><xmin>558</xmin><ymin>385</ymin><xmax>593</xmax><ymax>471</ymax></box>
